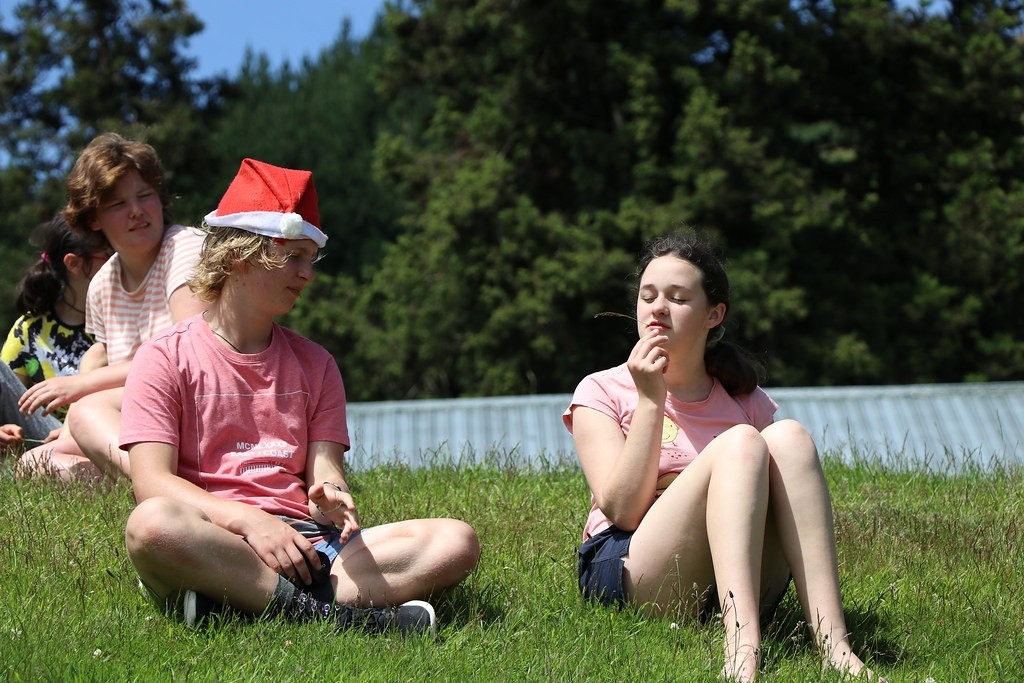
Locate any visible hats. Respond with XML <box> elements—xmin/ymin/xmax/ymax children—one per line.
<box><xmin>204</xmin><ymin>158</ymin><xmax>328</xmax><ymax>250</ymax></box>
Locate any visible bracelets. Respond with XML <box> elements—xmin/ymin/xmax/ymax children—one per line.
<box><xmin>317</xmin><ymin>481</ymin><xmax>342</xmax><ymax>518</ymax></box>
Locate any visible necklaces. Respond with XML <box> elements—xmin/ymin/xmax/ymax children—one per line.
<box><xmin>212</xmin><ymin>328</ymin><xmax>243</xmax><ymax>354</ymax></box>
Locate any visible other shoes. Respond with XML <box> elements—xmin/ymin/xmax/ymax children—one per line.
<box><xmin>184</xmin><ymin>589</ymin><xmax>255</xmax><ymax>629</ymax></box>
<box><xmin>343</xmin><ymin>598</ymin><xmax>437</xmax><ymax>638</ymax></box>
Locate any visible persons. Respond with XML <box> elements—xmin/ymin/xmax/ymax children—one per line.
<box><xmin>561</xmin><ymin>226</ymin><xmax>882</xmax><ymax>683</ymax></box>
<box><xmin>0</xmin><ymin>207</ymin><xmax>116</xmax><ymax>453</ymax></box>
<box><xmin>17</xmin><ymin>133</ymin><xmax>211</xmax><ymax>490</ymax></box>
<box><xmin>120</xmin><ymin>152</ymin><xmax>479</xmax><ymax>636</ymax></box>
